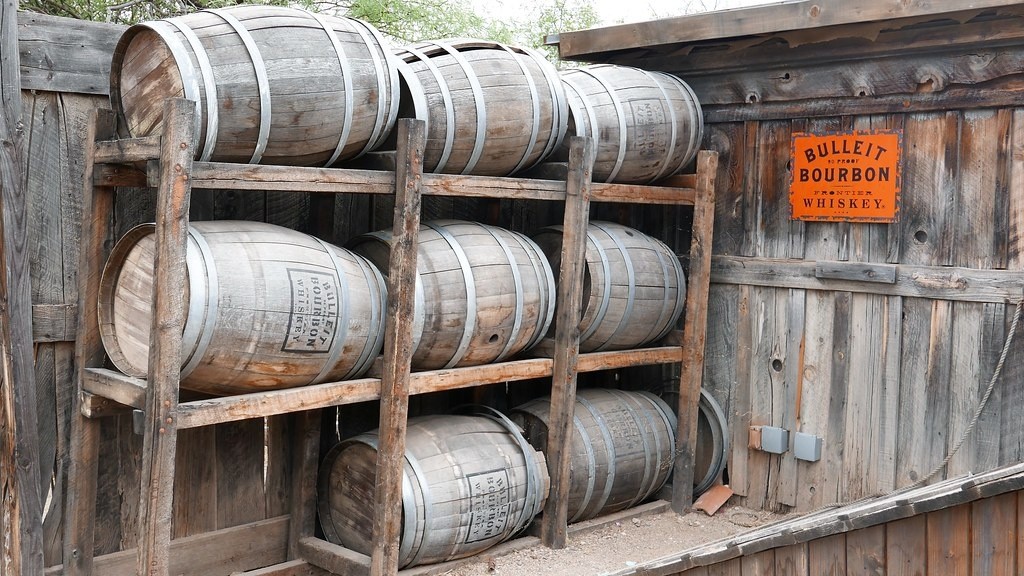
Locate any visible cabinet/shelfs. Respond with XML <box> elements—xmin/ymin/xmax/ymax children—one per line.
<box><xmin>64</xmin><ymin>95</ymin><xmax>719</xmax><ymax>576</ymax></box>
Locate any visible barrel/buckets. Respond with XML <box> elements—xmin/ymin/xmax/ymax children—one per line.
<box><xmin>110</xmin><ymin>5</ymin><xmax>401</xmax><ymax>165</ymax></box>
<box><xmin>528</xmin><ymin>220</ymin><xmax>686</xmax><ymax>350</ymax></box>
<box><xmin>343</xmin><ymin>220</ymin><xmax>555</xmax><ymax>368</ymax></box>
<box><xmin>555</xmin><ymin>65</ymin><xmax>705</xmax><ymax>184</ymax></box>
<box><xmin>391</xmin><ymin>39</ymin><xmax>569</xmax><ymax>176</ymax></box>
<box><xmin>317</xmin><ymin>405</ymin><xmax>545</xmax><ymax>570</ymax></box>
<box><xmin>97</xmin><ymin>221</ymin><xmax>387</xmax><ymax>399</ymax></box>
<box><xmin>506</xmin><ymin>390</ymin><xmax>676</xmax><ymax>523</ymax></box>
